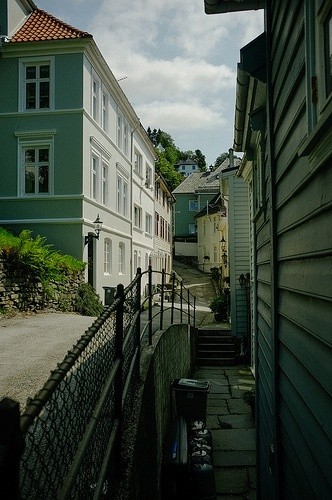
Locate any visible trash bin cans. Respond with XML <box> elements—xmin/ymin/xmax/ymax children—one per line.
<box><xmin>169</xmin><ymin>378</ymin><xmax>211</xmax><ymax>430</ymax></box>
<box><xmin>102</xmin><ymin>287</ymin><xmax>116</xmax><ymax>306</ymax></box>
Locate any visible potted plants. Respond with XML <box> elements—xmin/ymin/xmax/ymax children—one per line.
<box><xmin>209</xmin><ymin>298</ymin><xmax>229</xmax><ymax>322</ymax></box>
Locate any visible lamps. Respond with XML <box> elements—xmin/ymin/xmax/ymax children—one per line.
<box><xmin>86</xmin><ymin>214</ymin><xmax>104</xmax><ymax>244</ymax></box>
<box><xmin>220</xmin><ymin>236</ymin><xmax>229</xmax><ymax>253</ymax></box>
<box><xmin>221</xmin><ymin>253</ymin><xmax>228</xmax><ymax>268</ymax></box>
